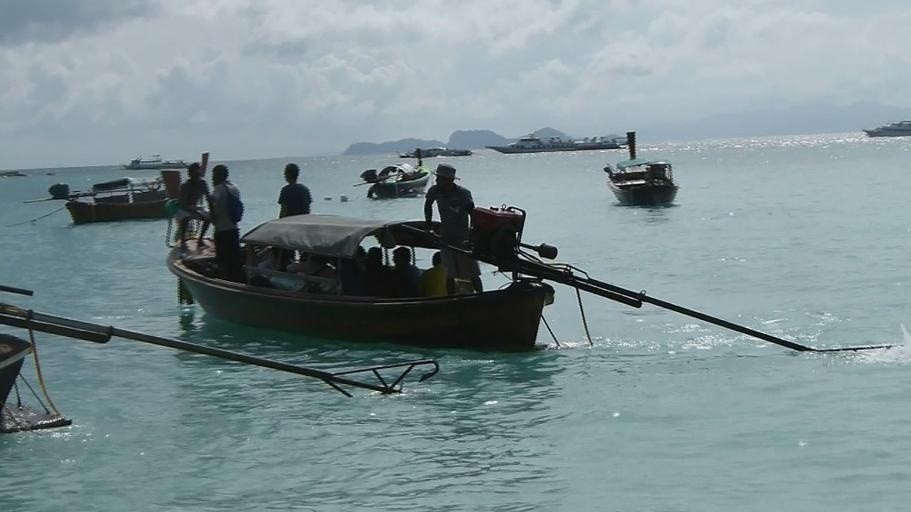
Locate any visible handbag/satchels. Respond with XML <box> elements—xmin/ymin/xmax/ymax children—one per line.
<box><xmin>223</xmin><ymin>183</ymin><xmax>246</xmax><ymax>223</ymax></box>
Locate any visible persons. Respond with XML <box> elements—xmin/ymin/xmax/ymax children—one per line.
<box><xmin>278</xmin><ymin>164</ymin><xmax>312</xmax><ymax>219</ymax></box>
<box><xmin>226</xmin><ymin>245</ymin><xmax>446</xmax><ymax>299</ymax></box>
<box><xmin>424</xmin><ymin>165</ymin><xmax>482</xmax><ymax>298</ymax></box>
<box><xmin>176</xmin><ymin>163</ymin><xmax>214</xmax><ymax>250</ymax></box>
<box><xmin>198</xmin><ymin>165</ymin><xmax>244</xmax><ymax>279</ymax></box>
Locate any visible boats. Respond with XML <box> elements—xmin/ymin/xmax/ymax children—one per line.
<box><xmin>1</xmin><ymin>271</ymin><xmax>447</xmax><ymax>442</ymax></box>
<box><xmin>159</xmin><ymin>198</ymin><xmax>813</xmax><ymax>360</ymax></box>
<box><xmin>862</xmin><ymin>118</ymin><xmax>911</xmax><ymax>140</ymax></box>
<box><xmin>402</xmin><ymin>145</ymin><xmax>473</xmax><ymax>157</ymax></box>
<box><xmin>356</xmin><ymin>147</ymin><xmax>432</xmax><ymax>201</ymax></box>
<box><xmin>120</xmin><ymin>153</ymin><xmax>196</xmax><ymax>170</ymax></box>
<box><xmin>604</xmin><ymin>130</ymin><xmax>681</xmax><ymax>208</ymax></box>
<box><xmin>23</xmin><ymin>167</ymin><xmax>214</xmax><ymax>225</ymax></box>
<box><xmin>484</xmin><ymin>130</ymin><xmax>622</xmax><ymax>154</ymax></box>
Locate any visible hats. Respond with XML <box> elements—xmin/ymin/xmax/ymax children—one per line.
<box><xmin>430</xmin><ymin>161</ymin><xmax>463</xmax><ymax>181</ymax></box>
